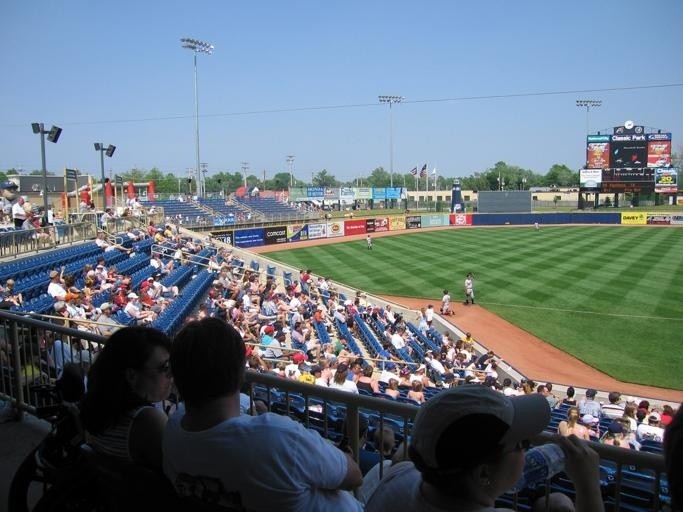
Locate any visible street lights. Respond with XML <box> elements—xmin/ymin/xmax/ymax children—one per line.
<box><xmin>184</xmin><ymin>167</ymin><xmax>194</xmax><ymax>193</ymax></box>
<box><xmin>31</xmin><ymin>122</ymin><xmax>61</xmax><ymax>233</ymax></box>
<box><xmin>285</xmin><ymin>154</ymin><xmax>296</xmax><ymax>185</ymax></box>
<box><xmin>180</xmin><ymin>36</ymin><xmax>214</xmax><ymax>196</ymax></box>
<box><xmin>575</xmin><ymin>98</ymin><xmax>602</xmax><ymax>136</ymax></box>
<box><xmin>375</xmin><ymin>94</ymin><xmax>404</xmax><ymax>185</ymax></box>
<box><xmin>93</xmin><ymin>142</ymin><xmax>116</xmax><ymax>211</ymax></box>
<box><xmin>240</xmin><ymin>160</ymin><xmax>250</xmax><ymax>187</ymax></box>
<box><xmin>200</xmin><ymin>162</ymin><xmax>209</xmax><ymax>198</ymax></box>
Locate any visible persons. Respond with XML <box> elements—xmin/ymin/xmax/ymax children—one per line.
<box><xmin>56</xmin><ymin>324</ymin><xmax>173</xmax><ymax>470</ymax></box>
<box><xmin>662</xmin><ymin>404</ymin><xmax>681</xmax><ymax>510</ymax></box>
<box><xmin>534</xmin><ymin>219</ymin><xmax>539</xmax><ymax>232</ymax></box>
<box><xmin>156</xmin><ymin>317</ymin><xmax>365</xmax><ymax>511</ymax></box>
<box><xmin>501</xmin><ymin>377</ymin><xmax>677</xmax><ymax>453</ymax></box>
<box><xmin>0</xmin><ymin>179</ymin><xmax>205</xmax><ymax>423</ymax></box>
<box><xmin>366</xmin><ymin>234</ymin><xmax>373</xmax><ymax>250</ymax></box>
<box><xmin>205</xmin><ymin>246</ymin><xmax>499</xmax><ymax>496</ymax></box>
<box><xmin>365</xmin><ymin>391</ymin><xmax>608</xmax><ymax>512</ymax></box>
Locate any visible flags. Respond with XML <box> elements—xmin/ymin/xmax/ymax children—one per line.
<box><xmin>419</xmin><ymin>163</ymin><xmax>427</xmax><ymax>178</ymax></box>
<box><xmin>409</xmin><ymin>166</ymin><xmax>417</xmax><ymax>177</ymax></box>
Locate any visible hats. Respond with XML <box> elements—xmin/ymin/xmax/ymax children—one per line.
<box><xmin>309</xmin><ymin>365</ymin><xmax>324</xmax><ymax>374</ymax></box>
<box><xmin>49</xmin><ymin>271</ymin><xmax>59</xmax><ymax>278</ymax></box>
<box><xmin>100</xmin><ymin>303</ymin><xmax>112</xmax><ymax>310</ymax></box>
<box><xmin>647</xmin><ymin>411</ymin><xmax>661</xmax><ymax>422</ymax></box>
<box><xmin>412</xmin><ymin>382</ymin><xmax>550</xmax><ymax>487</ymax></box>
<box><xmin>585</xmin><ymin>389</ymin><xmax>597</xmax><ymax>398</ymax></box>
<box><xmin>608</xmin><ymin>423</ymin><xmax>623</xmax><ymax>433</ymax></box>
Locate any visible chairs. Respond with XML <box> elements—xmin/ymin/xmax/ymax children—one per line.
<box><xmin>135</xmin><ymin>189</ymin><xmax>320</xmax><ymax>226</ymax></box>
<box><xmin>527</xmin><ymin>386</ymin><xmax>682</xmax><ymax>509</ymax></box>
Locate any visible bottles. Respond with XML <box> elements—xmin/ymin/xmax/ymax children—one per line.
<box><xmin>506</xmin><ymin>443</ymin><xmax>565</xmax><ymax>497</ymax></box>
